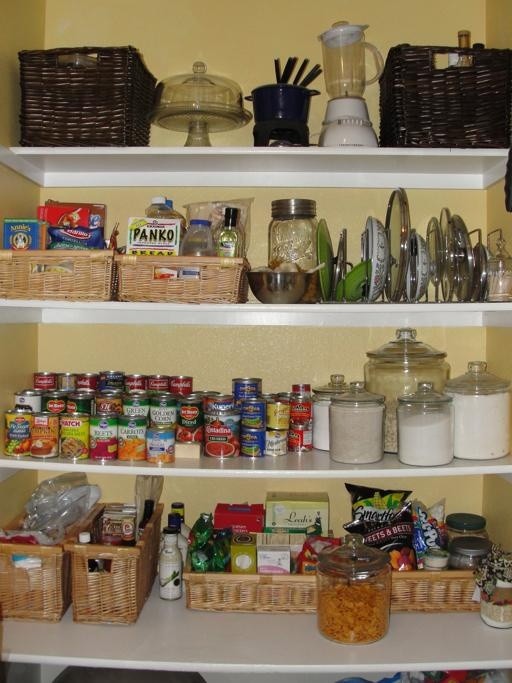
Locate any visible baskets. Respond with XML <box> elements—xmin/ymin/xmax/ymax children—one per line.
<box><xmin>182</xmin><ymin>548</ymin><xmax>487</xmax><ymax>612</ymax></box>
<box><xmin>18</xmin><ymin>47</ymin><xmax>157</xmax><ymax>147</ymax></box>
<box><xmin>0</xmin><ymin>503</ymin><xmax>101</xmax><ymax>622</ymax></box>
<box><xmin>379</xmin><ymin>45</ymin><xmax>510</xmax><ymax>148</ymax></box>
<box><xmin>64</xmin><ymin>504</ymin><xmax>164</xmax><ymax>626</ymax></box>
<box><xmin>114</xmin><ymin>255</ymin><xmax>250</xmax><ymax>304</ymax></box>
<box><xmin>0</xmin><ymin>250</ymin><xmax>115</xmax><ymax>302</ymax></box>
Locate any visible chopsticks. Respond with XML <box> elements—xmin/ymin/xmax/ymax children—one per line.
<box><xmin>273</xmin><ymin>56</ymin><xmax>322</xmax><ymax>88</ymax></box>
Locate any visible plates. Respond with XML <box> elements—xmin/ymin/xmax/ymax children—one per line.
<box><xmin>317</xmin><ymin>188</ymin><xmax>488</xmax><ymax>303</ymax></box>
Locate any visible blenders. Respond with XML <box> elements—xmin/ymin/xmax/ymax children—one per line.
<box><xmin>316</xmin><ymin>20</ymin><xmax>385</xmax><ymax>147</ymax></box>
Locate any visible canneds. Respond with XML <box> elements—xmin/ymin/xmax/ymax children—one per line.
<box><xmin>205</xmin><ymin>375</ymin><xmax>314</xmax><ymax>460</ymax></box>
<box><xmin>444</xmin><ymin>512</ymin><xmax>489</xmax><ymax>548</ymax></box>
<box><xmin>2</xmin><ymin>370</ymin><xmax>206</xmax><ymax>465</ymax></box>
<box><xmin>447</xmin><ymin>535</ymin><xmax>493</xmax><ymax>571</ymax></box>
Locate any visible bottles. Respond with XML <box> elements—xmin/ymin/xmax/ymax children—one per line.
<box><xmin>167</xmin><ymin>503</ymin><xmax>191</xmax><ymax>541</ymax></box>
<box><xmin>453</xmin><ymin>30</ymin><xmax>473</xmax><ymax>68</ymax></box>
<box><xmin>145</xmin><ymin>194</ymin><xmax>176</xmax><ymax>217</ymax></box>
<box><xmin>137</xmin><ymin>499</ymin><xmax>154</xmax><ymax>538</ymax></box>
<box><xmin>478</xmin><ymin>577</ymin><xmax>512</xmax><ymax>631</ymax></box>
<box><xmin>156</xmin><ymin>514</ymin><xmax>188</xmax><ymax>565</ymax></box>
<box><xmin>488</xmin><ymin>237</ymin><xmax>512</xmax><ymax>301</ymax></box>
<box><xmin>267</xmin><ymin>197</ymin><xmax>317</xmax><ymax>270</ymax></box>
<box><xmin>423</xmin><ymin>512</ymin><xmax>492</xmax><ymax>571</ymax></box>
<box><xmin>79</xmin><ymin>531</ymin><xmax>98</xmax><ymax>571</ymax></box>
<box><xmin>316</xmin><ymin>532</ymin><xmax>393</xmax><ymax>647</ymax></box>
<box><xmin>214</xmin><ymin>207</ymin><xmax>243</xmax><ymax>256</ymax></box>
<box><xmin>120</xmin><ymin>516</ymin><xmax>137</xmax><ymax>547</ymax></box>
<box><xmin>312</xmin><ymin>326</ymin><xmax>512</xmax><ymax>466</ymax></box>
<box><xmin>178</xmin><ymin>218</ymin><xmax>214</xmax><ymax>279</ymax></box>
<box><xmin>158</xmin><ymin>526</ymin><xmax>183</xmax><ymax>600</ymax></box>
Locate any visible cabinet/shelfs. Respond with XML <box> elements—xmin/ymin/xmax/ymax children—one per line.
<box><xmin>1</xmin><ymin>148</ymin><xmax>512</xmax><ymax>683</ymax></box>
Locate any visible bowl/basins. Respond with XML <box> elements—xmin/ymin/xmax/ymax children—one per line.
<box><xmin>245</xmin><ymin>270</ymin><xmax>312</xmax><ymax>303</ymax></box>
